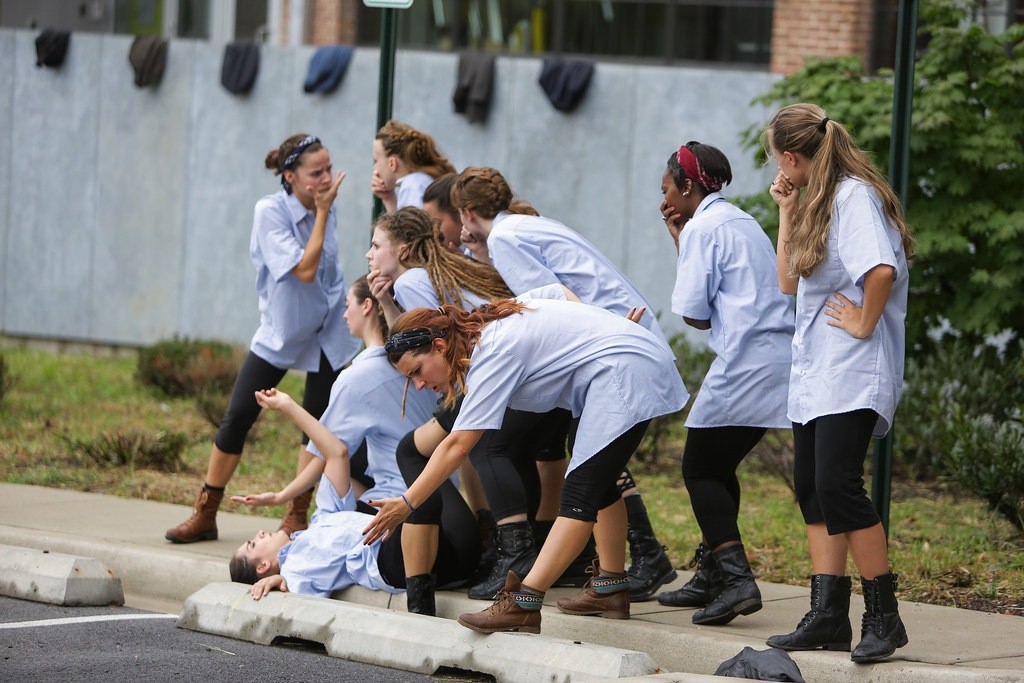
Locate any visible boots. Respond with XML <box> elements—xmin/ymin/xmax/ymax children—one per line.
<box><xmin>468</xmin><ymin>521</ymin><xmax>538</xmax><ymax>600</ymax></box>
<box><xmin>850</xmin><ymin>570</ymin><xmax>909</xmax><ymax>662</ymax></box>
<box><xmin>278</xmin><ymin>490</ymin><xmax>313</xmax><ymax>537</ymax></box>
<box><xmin>691</xmin><ymin>543</ymin><xmax>763</xmax><ymax>625</ymax></box>
<box><xmin>166</xmin><ymin>487</ymin><xmax>224</xmax><ymax>542</ymax></box>
<box><xmin>550</xmin><ymin>533</ymin><xmax>599</xmax><ymax>588</ymax></box>
<box><xmin>765</xmin><ymin>573</ymin><xmax>852</xmax><ymax>651</ymax></box>
<box><xmin>405</xmin><ymin>573</ymin><xmax>436</xmax><ymax>617</ymax></box>
<box><xmin>658</xmin><ymin>542</ymin><xmax>722</xmax><ymax>607</ymax></box>
<box><xmin>623</xmin><ymin>494</ymin><xmax>678</xmax><ymax>601</ymax></box>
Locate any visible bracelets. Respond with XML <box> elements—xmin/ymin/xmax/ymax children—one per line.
<box><xmin>402</xmin><ymin>495</ymin><xmax>415</xmax><ymax>512</ymax></box>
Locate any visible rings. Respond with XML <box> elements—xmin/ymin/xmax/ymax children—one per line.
<box><xmin>772</xmin><ymin>181</ymin><xmax>775</xmax><ymax>185</ymax></box>
<box><xmin>663</xmin><ymin>216</ymin><xmax>668</xmax><ymax>220</ymax></box>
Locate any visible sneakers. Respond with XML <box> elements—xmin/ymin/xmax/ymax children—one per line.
<box><xmin>557</xmin><ymin>558</ymin><xmax>630</xmax><ymax>620</ymax></box>
<box><xmin>457</xmin><ymin>570</ymin><xmax>542</xmax><ymax>634</ymax></box>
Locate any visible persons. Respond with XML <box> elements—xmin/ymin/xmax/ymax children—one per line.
<box><xmin>453</xmin><ymin>166</ymin><xmax>677</xmax><ymax>600</ymax></box>
<box><xmin>658</xmin><ymin>141</ymin><xmax>794</xmax><ymax>624</ymax></box>
<box><xmin>165</xmin><ymin>134</ymin><xmax>362</xmax><ymax>543</ymax></box>
<box><xmin>229</xmin><ymin>387</ymin><xmax>465</xmax><ymax>617</ymax></box>
<box><xmin>363</xmin><ymin>284</ymin><xmax>690</xmax><ymax>634</ymax></box>
<box><xmin>766</xmin><ymin>102</ymin><xmax>908</xmax><ymax>664</ymax></box>
<box><xmin>233</xmin><ymin>120</ymin><xmax>599</xmax><ymax>597</ymax></box>
